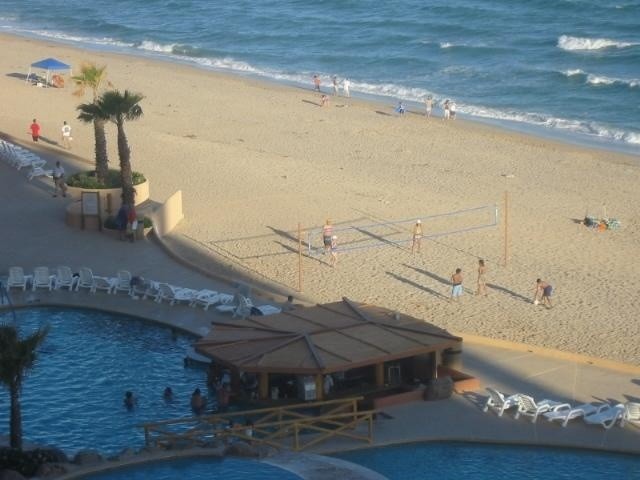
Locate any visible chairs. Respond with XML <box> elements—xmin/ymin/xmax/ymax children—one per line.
<box><xmin>483</xmin><ymin>386</ymin><xmax>640</xmax><ymax>431</ymax></box>
<box><xmin>0</xmin><ymin>137</ymin><xmax>53</xmax><ymax>183</ymax></box>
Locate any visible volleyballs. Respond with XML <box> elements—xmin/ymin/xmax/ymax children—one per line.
<box><xmin>533</xmin><ymin>300</ymin><xmax>539</xmax><ymax>306</ymax></box>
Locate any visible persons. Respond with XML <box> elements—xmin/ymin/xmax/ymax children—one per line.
<box><xmin>162</xmin><ymin>387</ymin><xmax>174</xmax><ymax>402</ymax></box>
<box><xmin>224</xmin><ymin>421</ymin><xmax>253</xmax><ymax>449</ymax></box>
<box><xmin>321</xmin><ymin>219</ymin><xmax>338</xmax><ymax>269</ymax></box>
<box><xmin>61</xmin><ymin>121</ymin><xmax>72</xmax><ymax>150</ymax></box>
<box><xmin>30</xmin><ymin>118</ymin><xmax>40</xmax><ymax>142</ymax></box>
<box><xmin>396</xmin><ymin>96</ymin><xmax>458</xmax><ymax>121</ymax></box>
<box><xmin>116</xmin><ymin>199</ymin><xmax>138</xmax><ymax>242</ymax></box>
<box><xmin>411</xmin><ymin>219</ymin><xmax>422</xmax><ymax>254</ymax></box>
<box><xmin>282</xmin><ymin>296</ymin><xmax>298</xmax><ymax>313</ymax></box>
<box><xmin>450</xmin><ymin>268</ymin><xmax>464</xmax><ymax>309</ymax></box>
<box><xmin>123</xmin><ymin>391</ymin><xmax>137</xmax><ymax>411</ymax></box>
<box><xmin>51</xmin><ymin>160</ymin><xmax>68</xmax><ymax>199</ymax></box>
<box><xmin>533</xmin><ymin>278</ymin><xmax>554</xmax><ymax>309</ymax></box>
<box><xmin>313</xmin><ymin>74</ymin><xmax>351</xmax><ymax>107</ymax></box>
<box><xmin>476</xmin><ymin>259</ymin><xmax>489</xmax><ymax>297</ymax></box>
<box><xmin>191</xmin><ymin>374</ymin><xmax>259</xmax><ymax>416</ymax></box>
